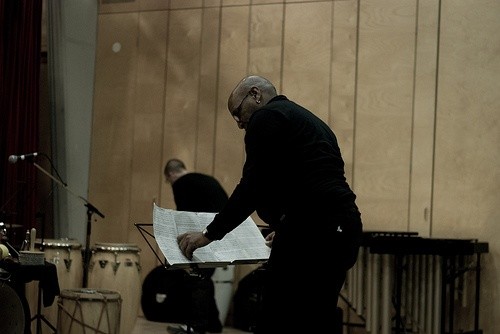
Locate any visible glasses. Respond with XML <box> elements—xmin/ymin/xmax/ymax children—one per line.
<box><xmin>232</xmin><ymin>90</ymin><xmax>252</xmax><ymax>123</ymax></box>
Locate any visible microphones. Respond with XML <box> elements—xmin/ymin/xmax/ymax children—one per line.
<box><xmin>8</xmin><ymin>152</ymin><xmax>41</xmax><ymax>164</ymax></box>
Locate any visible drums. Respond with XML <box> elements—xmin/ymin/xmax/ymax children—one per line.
<box><xmin>88</xmin><ymin>241</ymin><xmax>142</xmax><ymax>334</ymax></box>
<box><xmin>0</xmin><ymin>283</ymin><xmax>30</xmax><ymax>334</ymax></box>
<box><xmin>26</xmin><ymin>238</ymin><xmax>85</xmax><ymax>334</ymax></box>
<box><xmin>57</xmin><ymin>289</ymin><xmax>122</xmax><ymax>334</ymax></box>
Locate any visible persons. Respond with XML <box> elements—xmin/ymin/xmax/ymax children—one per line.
<box><xmin>176</xmin><ymin>75</ymin><xmax>362</xmax><ymax>334</ymax></box>
<box><xmin>163</xmin><ymin>157</ymin><xmax>230</xmax><ymax>277</ymax></box>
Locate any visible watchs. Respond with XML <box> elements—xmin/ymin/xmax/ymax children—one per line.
<box><xmin>202</xmin><ymin>228</ymin><xmax>214</xmax><ymax>241</ymax></box>
